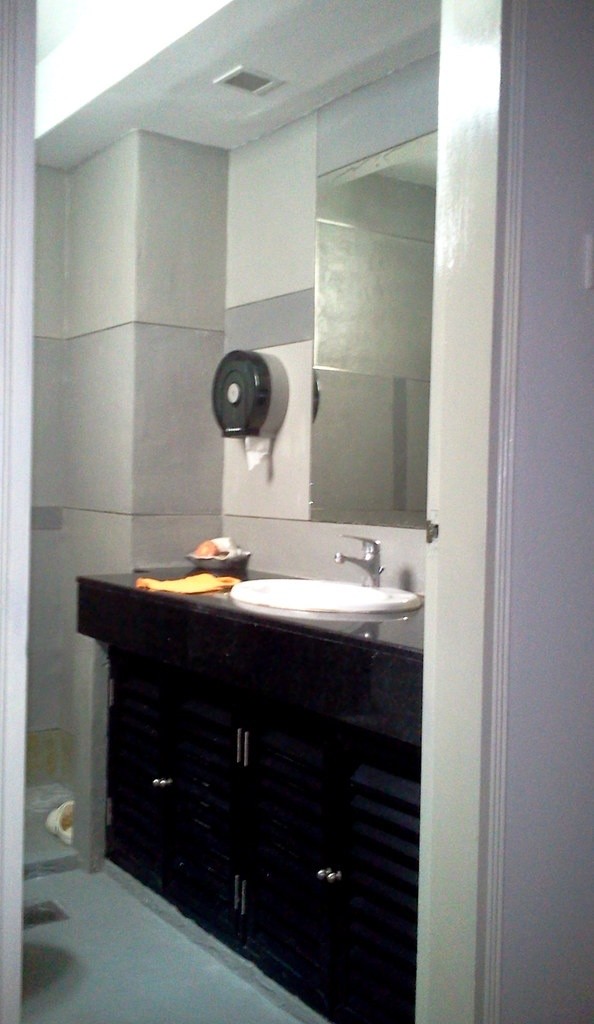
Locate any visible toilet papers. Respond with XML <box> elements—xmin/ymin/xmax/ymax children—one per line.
<box><xmin>210</xmin><ymin>350</ymin><xmax>289</xmax><ymax>471</ymax></box>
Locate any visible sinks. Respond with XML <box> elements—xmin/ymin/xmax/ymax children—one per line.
<box><xmin>231</xmin><ymin>578</ymin><xmax>422</xmax><ymax>612</ymax></box>
<box><xmin>239</xmin><ymin>604</ymin><xmax>410</xmax><ymax>624</ymax></box>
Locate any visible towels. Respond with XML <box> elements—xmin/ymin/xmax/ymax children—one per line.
<box><xmin>135</xmin><ymin>572</ymin><xmax>243</xmax><ymax>594</ymax></box>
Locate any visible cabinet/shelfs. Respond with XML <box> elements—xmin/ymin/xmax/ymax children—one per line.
<box><xmin>97</xmin><ymin>643</ymin><xmax>418</xmax><ymax>1023</ymax></box>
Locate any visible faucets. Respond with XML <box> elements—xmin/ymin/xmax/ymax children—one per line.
<box><xmin>334</xmin><ymin>533</ymin><xmax>383</xmax><ymax>588</ymax></box>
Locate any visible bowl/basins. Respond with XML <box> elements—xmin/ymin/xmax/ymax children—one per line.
<box><xmin>185</xmin><ymin>551</ymin><xmax>253</xmax><ymax>571</ymax></box>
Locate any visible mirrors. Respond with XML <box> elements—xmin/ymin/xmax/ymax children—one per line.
<box><xmin>304</xmin><ymin>130</ymin><xmax>438</xmax><ymax>530</ymax></box>
